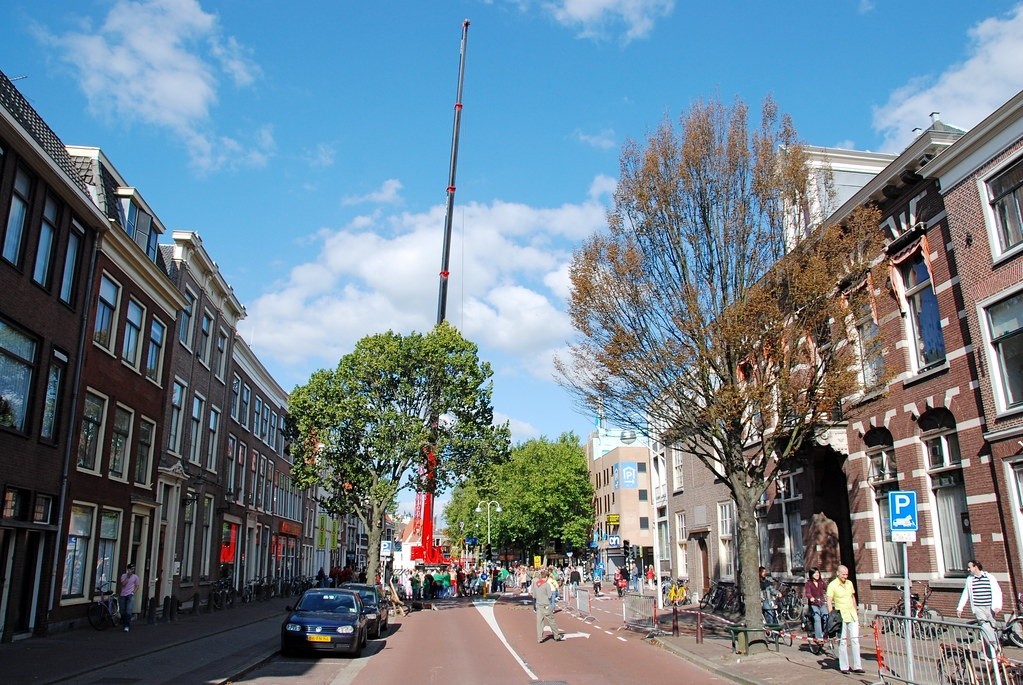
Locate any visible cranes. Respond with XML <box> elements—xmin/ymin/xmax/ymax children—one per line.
<box><xmin>395</xmin><ymin>18</ymin><xmax>475</xmax><ymax>607</ymax></box>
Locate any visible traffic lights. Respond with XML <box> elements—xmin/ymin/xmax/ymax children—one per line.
<box><xmin>484</xmin><ymin>543</ymin><xmax>491</xmax><ymax>560</ymax></box>
<box><xmin>623</xmin><ymin>540</ymin><xmax>630</xmax><ymax>557</ymax></box>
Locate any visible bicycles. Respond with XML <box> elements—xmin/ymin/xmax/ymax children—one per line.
<box><xmin>762</xmin><ymin>576</ymin><xmax>842</xmax><ymax>659</ymax></box>
<box><xmin>697</xmin><ymin>576</ymin><xmax>745</xmax><ymax>617</ymax></box>
<box><xmin>879</xmin><ymin>579</ymin><xmax>1023</xmax><ymax>685</ymax></box>
<box><xmin>661</xmin><ymin>575</ymin><xmax>692</xmax><ymax>608</ymax></box>
<box><xmin>86</xmin><ymin>580</ymin><xmax>122</xmax><ymax>632</ymax></box>
<box><xmin>211</xmin><ymin>577</ymin><xmax>234</xmax><ymax>610</ymax></box>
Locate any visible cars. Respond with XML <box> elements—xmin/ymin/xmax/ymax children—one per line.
<box><xmin>280</xmin><ymin>586</ymin><xmax>372</xmax><ymax>659</ymax></box>
<box><xmin>241</xmin><ymin>575</ymin><xmax>390</xmax><ymax>640</ymax></box>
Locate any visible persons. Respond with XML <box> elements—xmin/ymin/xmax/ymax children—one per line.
<box><xmin>120</xmin><ymin>563</ymin><xmax>139</xmax><ymax>632</ymax></box>
<box><xmin>509</xmin><ymin>565</ymin><xmax>571</xmax><ymax>588</ymax></box>
<box><xmin>570</xmin><ymin>567</ymin><xmax>581</xmax><ymax>597</ymax></box>
<box><xmin>805</xmin><ymin>567</ymin><xmax>829</xmax><ymax>638</ymax></box>
<box><xmin>405</xmin><ymin>563</ymin><xmax>509</xmax><ymax>600</ymax></box>
<box><xmin>957</xmin><ymin>560</ymin><xmax>1003</xmax><ymax>661</ymax></box>
<box><xmin>644</xmin><ymin>565</ymin><xmax>655</xmax><ymax>585</ymax></box>
<box><xmin>632</xmin><ymin>561</ymin><xmax>639</xmax><ymax>592</ymax></box>
<box><xmin>530</xmin><ymin>571</ymin><xmax>564</xmax><ymax>643</ymax></box>
<box><xmin>759</xmin><ymin>566</ymin><xmax>781</xmax><ymax>623</ymax></box>
<box><xmin>318</xmin><ymin>567</ymin><xmax>326</xmax><ymax>587</ymax></box>
<box><xmin>329</xmin><ymin>566</ymin><xmax>368</xmax><ymax>588</ymax></box>
<box><xmin>531</xmin><ymin>570</ymin><xmax>559</xmax><ymax>613</ymax></box>
<box><xmin>620</xmin><ymin>565</ymin><xmax>630</xmax><ymax>592</ymax></box>
<box><xmin>826</xmin><ymin>565</ymin><xmax>866</xmax><ymax>675</ymax></box>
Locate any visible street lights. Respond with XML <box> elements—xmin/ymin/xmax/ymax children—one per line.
<box><xmin>475</xmin><ymin>500</ymin><xmax>503</xmax><ymax>593</ymax></box>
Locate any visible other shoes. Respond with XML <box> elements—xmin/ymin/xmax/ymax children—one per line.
<box><xmin>554</xmin><ymin>634</ymin><xmax>562</xmax><ymax>641</ymax></box>
<box><xmin>853</xmin><ymin>669</ymin><xmax>865</xmax><ymax>673</ymax></box>
<box><xmin>538</xmin><ymin>639</ymin><xmax>544</xmax><ymax>643</ymax></box>
<box><xmin>841</xmin><ymin>670</ymin><xmax>850</xmax><ymax>674</ymax></box>
